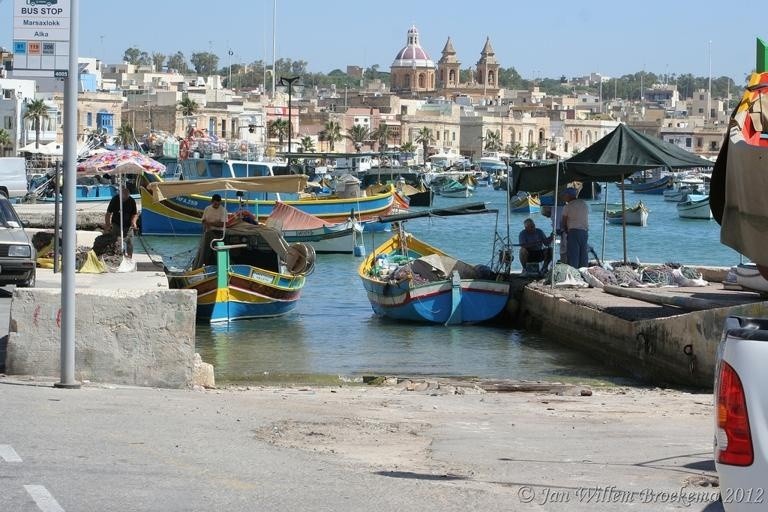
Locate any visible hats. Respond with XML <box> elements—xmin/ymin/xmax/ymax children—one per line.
<box><xmin>560</xmin><ymin>187</ymin><xmax>577</xmax><ymax>196</ymax></box>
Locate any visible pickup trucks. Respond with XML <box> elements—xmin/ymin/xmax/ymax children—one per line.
<box><xmin>712</xmin><ymin>311</ymin><xmax>767</xmax><ymax>512</ymax></box>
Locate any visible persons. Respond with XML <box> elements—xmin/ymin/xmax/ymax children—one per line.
<box><xmin>517</xmin><ymin>219</ymin><xmax>555</xmax><ymax>277</ymax></box>
<box><xmin>105</xmin><ymin>188</ymin><xmax>140</xmax><ymax>259</ymax></box>
<box><xmin>559</xmin><ymin>186</ymin><xmax>592</xmax><ymax>268</ymax></box>
<box><xmin>540</xmin><ymin>205</ymin><xmax>569</xmax><ymax>264</ymax></box>
<box><xmin>200</xmin><ymin>194</ymin><xmax>226</xmax><ymax>230</ymax></box>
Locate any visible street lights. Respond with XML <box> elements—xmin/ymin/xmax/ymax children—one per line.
<box><xmin>707</xmin><ymin>40</ymin><xmax>712</xmax><ymax>121</ymax></box>
<box><xmin>277</xmin><ymin>74</ymin><xmax>303</xmax><ymax>151</ymax></box>
<box><xmin>228</xmin><ymin>47</ymin><xmax>235</xmax><ymax>86</ymax></box>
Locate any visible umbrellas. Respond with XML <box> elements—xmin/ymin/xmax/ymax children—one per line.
<box><xmin>77</xmin><ymin>148</ymin><xmax>168</xmax><ymax>256</ymax></box>
<box><xmin>16</xmin><ymin>141</ymin><xmax>47</xmax><ymax>167</ymax></box>
<box><xmin>38</xmin><ymin>141</ymin><xmax>64</xmax><ymax>165</ymax></box>
<box><xmin>512</xmin><ymin>123</ymin><xmax>714</xmax><ymax>261</ymax></box>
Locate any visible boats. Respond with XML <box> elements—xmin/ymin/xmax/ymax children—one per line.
<box><xmin>617</xmin><ymin>169</ymin><xmax>713</xmax><ymax>201</ymax></box>
<box><xmin>604</xmin><ymin>201</ymin><xmax>651</xmax><ymax>227</ymax></box>
<box><xmin>708</xmin><ymin>37</ymin><xmax>767</xmax><ymax>284</ymax></box>
<box><xmin>166</xmin><ymin>221</ymin><xmax>308</xmax><ymax>326</ymax></box>
<box><xmin>354</xmin><ymin>227</ymin><xmax>510</xmax><ymax>329</ymax></box>
<box><xmin>9</xmin><ymin>128</ymin><xmax>600</xmax><ymax>238</ymax></box>
<box><xmin>677</xmin><ymin>192</ymin><xmax>714</xmax><ymax>220</ymax></box>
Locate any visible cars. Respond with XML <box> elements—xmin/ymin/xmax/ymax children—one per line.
<box><xmin>0</xmin><ymin>184</ymin><xmax>45</xmax><ymax>288</ymax></box>
